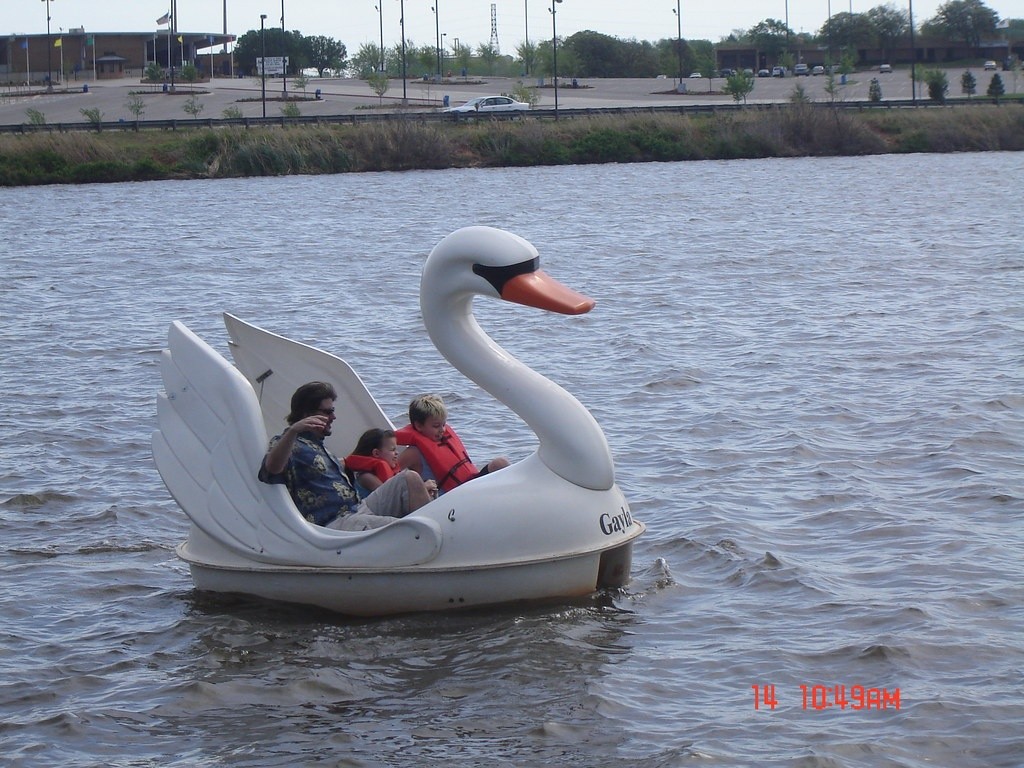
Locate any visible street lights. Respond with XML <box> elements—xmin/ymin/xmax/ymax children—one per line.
<box><xmin>260</xmin><ymin>14</ymin><xmax>267</xmax><ymax>119</ymax></box>
<box><xmin>548</xmin><ymin>0</ymin><xmax>563</xmax><ymax>118</ymax></box>
<box><xmin>441</xmin><ymin>34</ymin><xmax>447</xmax><ymax>77</ymax></box>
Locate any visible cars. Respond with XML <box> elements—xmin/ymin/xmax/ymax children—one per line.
<box><xmin>794</xmin><ymin>64</ymin><xmax>809</xmax><ymax>76</ymax></box>
<box><xmin>443</xmin><ymin>95</ymin><xmax>531</xmax><ymax>121</ymax></box>
<box><xmin>812</xmin><ymin>66</ymin><xmax>824</xmax><ymax>76</ymax></box>
<box><xmin>690</xmin><ymin>72</ymin><xmax>702</xmax><ymax>78</ymax></box>
<box><xmin>758</xmin><ymin>70</ymin><xmax>770</xmax><ymax>78</ymax></box>
<box><xmin>879</xmin><ymin>64</ymin><xmax>891</xmax><ymax>73</ymax></box>
<box><xmin>743</xmin><ymin>69</ymin><xmax>755</xmax><ymax>77</ymax></box>
<box><xmin>657</xmin><ymin>75</ymin><xmax>668</xmax><ymax>79</ymax></box>
<box><xmin>720</xmin><ymin>68</ymin><xmax>736</xmax><ymax>78</ymax></box>
<box><xmin>772</xmin><ymin>67</ymin><xmax>786</xmax><ymax>76</ymax></box>
<box><xmin>984</xmin><ymin>61</ymin><xmax>997</xmax><ymax>71</ymax></box>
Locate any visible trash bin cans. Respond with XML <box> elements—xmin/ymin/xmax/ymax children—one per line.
<box><xmin>573</xmin><ymin>78</ymin><xmax>577</xmax><ymax>87</ymax></box>
<box><xmin>83</xmin><ymin>85</ymin><xmax>88</xmax><ymax>92</ymax></box>
<box><xmin>316</xmin><ymin>89</ymin><xmax>321</xmax><ymax>98</ymax></box>
<box><xmin>841</xmin><ymin>73</ymin><xmax>846</xmax><ymax>84</ymax></box>
<box><xmin>119</xmin><ymin>118</ymin><xmax>125</xmax><ymax>122</ymax></box>
<box><xmin>444</xmin><ymin>96</ymin><xmax>449</xmax><ymax>107</ymax></box>
<box><xmin>162</xmin><ymin>83</ymin><xmax>168</xmax><ymax>90</ymax></box>
<box><xmin>448</xmin><ymin>70</ymin><xmax>452</xmax><ymax>76</ymax></box>
<box><xmin>462</xmin><ymin>69</ymin><xmax>466</xmax><ymax>76</ymax></box>
<box><xmin>238</xmin><ymin>71</ymin><xmax>243</xmax><ymax>78</ymax></box>
<box><xmin>423</xmin><ymin>74</ymin><xmax>428</xmax><ymax>81</ymax></box>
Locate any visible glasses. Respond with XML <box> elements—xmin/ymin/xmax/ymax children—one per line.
<box><xmin>318</xmin><ymin>407</ymin><xmax>334</xmax><ymax>415</ymax></box>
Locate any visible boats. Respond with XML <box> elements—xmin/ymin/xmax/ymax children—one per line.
<box><xmin>148</xmin><ymin>224</ymin><xmax>644</xmax><ymax>618</ymax></box>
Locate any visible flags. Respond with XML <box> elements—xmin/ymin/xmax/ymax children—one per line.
<box><xmin>86</xmin><ymin>38</ymin><xmax>93</xmax><ymax>45</ymax></box>
<box><xmin>21</xmin><ymin>41</ymin><xmax>27</xmax><ymax>48</ymax></box>
<box><xmin>156</xmin><ymin>13</ymin><xmax>168</xmax><ymax>25</ymax></box>
<box><xmin>178</xmin><ymin>36</ymin><xmax>182</xmax><ymax>43</ymax></box>
<box><xmin>54</xmin><ymin>39</ymin><xmax>61</xmax><ymax>47</ymax></box>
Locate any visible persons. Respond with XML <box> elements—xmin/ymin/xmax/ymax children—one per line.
<box><xmin>398</xmin><ymin>395</ymin><xmax>511</xmax><ymax>496</ymax></box>
<box><xmin>257</xmin><ymin>382</ymin><xmax>431</xmax><ymax>531</ymax></box>
<box><xmin>351</xmin><ymin>428</ymin><xmax>439</xmax><ymax>498</ymax></box>
